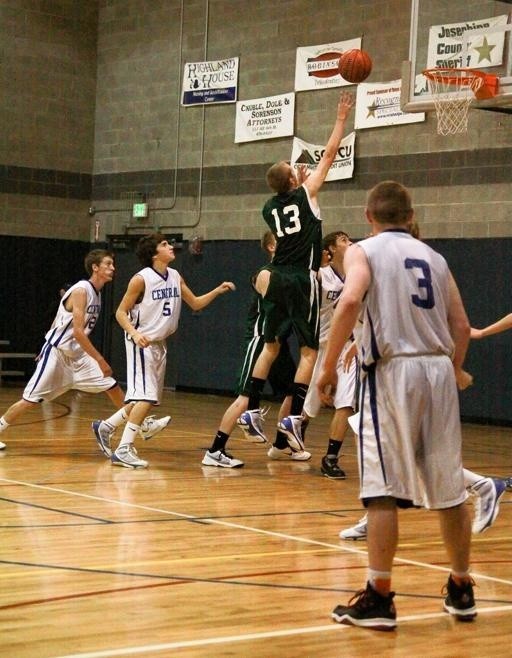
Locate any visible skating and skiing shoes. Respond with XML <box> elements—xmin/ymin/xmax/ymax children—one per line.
<box><xmin>472</xmin><ymin>479</ymin><xmax>506</xmax><ymax>533</ymax></box>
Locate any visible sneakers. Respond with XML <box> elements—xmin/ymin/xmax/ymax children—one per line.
<box><xmin>340</xmin><ymin>513</ymin><xmax>367</xmax><ymax>540</ymax></box>
<box><xmin>443</xmin><ymin>575</ymin><xmax>477</xmax><ymax>620</ymax></box>
<box><xmin>321</xmin><ymin>456</ymin><xmax>346</xmax><ymax>479</ymax></box>
<box><xmin>202</xmin><ymin>449</ymin><xmax>244</xmax><ymax>468</ymax></box>
<box><xmin>111</xmin><ymin>444</ymin><xmax>148</xmax><ymax>468</ymax></box>
<box><xmin>138</xmin><ymin>415</ymin><xmax>171</xmax><ymax>440</ymax></box>
<box><xmin>277</xmin><ymin>417</ymin><xmax>305</xmax><ymax>451</ymax></box>
<box><xmin>237</xmin><ymin>412</ymin><xmax>267</xmax><ymax>443</ymax></box>
<box><xmin>92</xmin><ymin>420</ymin><xmax>115</xmax><ymax>459</ymax></box>
<box><xmin>267</xmin><ymin>446</ymin><xmax>311</xmax><ymax>461</ymax></box>
<box><xmin>331</xmin><ymin>581</ymin><xmax>398</xmax><ymax>629</ymax></box>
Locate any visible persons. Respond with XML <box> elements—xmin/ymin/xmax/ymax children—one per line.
<box><xmin>0</xmin><ymin>248</ymin><xmax>172</xmax><ymax>449</ymax></box>
<box><xmin>234</xmin><ymin>230</ymin><xmax>309</xmax><ymax>444</ymax></box>
<box><xmin>471</xmin><ymin>313</ymin><xmax>510</xmax><ymax>339</ymax></box>
<box><xmin>338</xmin><ymin>220</ymin><xmax>512</xmax><ymax>541</ymax></box>
<box><xmin>298</xmin><ymin>231</ymin><xmax>358</xmax><ymax>481</ymax></box>
<box><xmin>201</xmin><ymin>90</ymin><xmax>356</xmax><ymax>469</ymax></box>
<box><xmin>91</xmin><ymin>236</ymin><xmax>236</xmax><ymax>469</ymax></box>
<box><xmin>316</xmin><ymin>181</ymin><xmax>476</xmax><ymax>632</ymax></box>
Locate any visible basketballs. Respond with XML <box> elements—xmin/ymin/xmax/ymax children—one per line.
<box><xmin>339</xmin><ymin>48</ymin><xmax>372</xmax><ymax>82</ymax></box>
